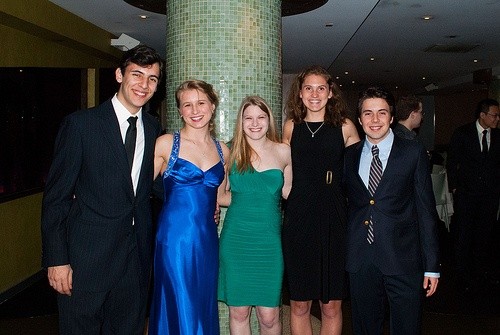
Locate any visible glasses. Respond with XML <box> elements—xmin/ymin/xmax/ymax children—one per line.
<box><xmin>487</xmin><ymin>113</ymin><xmax>500</xmax><ymax>117</ymax></box>
<box><xmin>415</xmin><ymin>110</ymin><xmax>425</xmax><ymax>116</ymax></box>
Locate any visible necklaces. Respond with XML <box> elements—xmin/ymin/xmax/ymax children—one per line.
<box><xmin>305</xmin><ymin>119</ymin><xmax>326</xmax><ymax>138</ymax></box>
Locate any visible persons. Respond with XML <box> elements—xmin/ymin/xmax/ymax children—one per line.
<box><xmin>444</xmin><ymin>99</ymin><xmax>500</xmax><ymax>290</ymax></box>
<box><xmin>153</xmin><ymin>80</ymin><xmax>232</xmax><ymax>335</ymax></box>
<box><xmin>282</xmin><ymin>65</ymin><xmax>361</xmax><ymax>335</ymax></box>
<box><xmin>41</xmin><ymin>47</ymin><xmax>221</xmax><ymax>335</ymax></box>
<box><xmin>218</xmin><ymin>95</ymin><xmax>292</xmax><ymax>335</ymax></box>
<box><xmin>343</xmin><ymin>88</ymin><xmax>441</xmax><ymax>335</ymax></box>
<box><xmin>395</xmin><ymin>93</ymin><xmax>424</xmax><ymax>138</ymax></box>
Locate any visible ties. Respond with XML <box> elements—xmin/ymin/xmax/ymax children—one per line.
<box><xmin>482</xmin><ymin>130</ymin><xmax>488</xmax><ymax>153</ymax></box>
<box><xmin>124</xmin><ymin>116</ymin><xmax>139</xmax><ymax>172</ymax></box>
<box><xmin>366</xmin><ymin>145</ymin><xmax>383</xmax><ymax>244</ymax></box>
